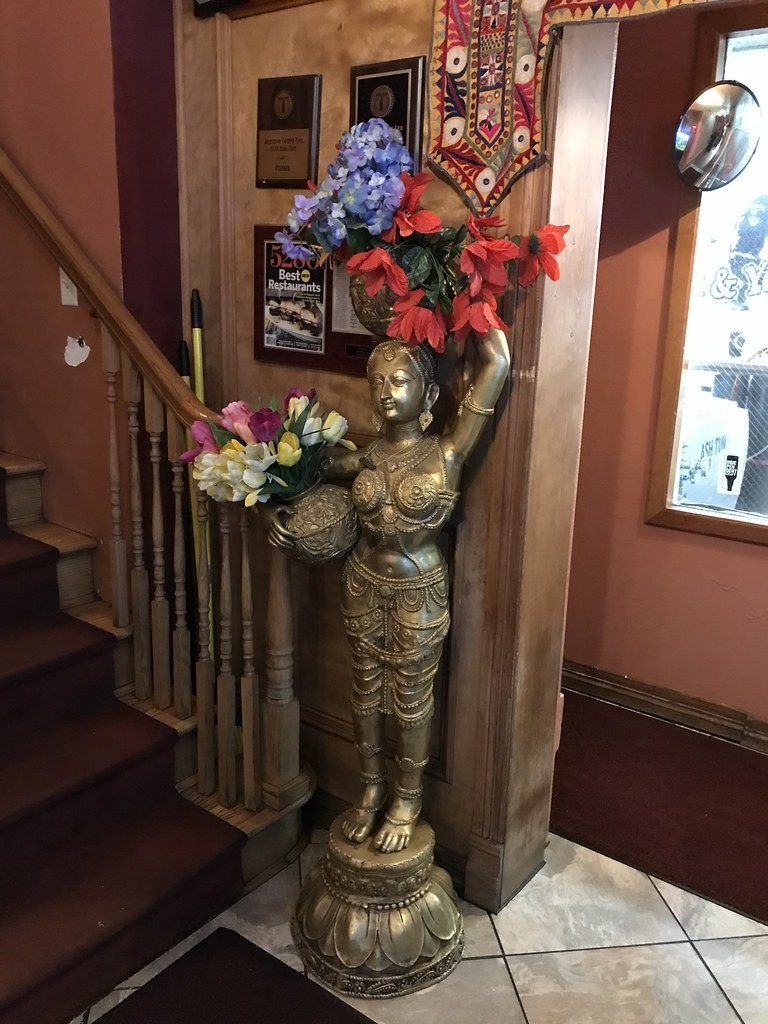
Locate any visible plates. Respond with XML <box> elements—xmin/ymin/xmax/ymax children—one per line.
<box><xmin>265</xmin><ymin>306</ymin><xmax>322</xmax><ymax>344</ymax></box>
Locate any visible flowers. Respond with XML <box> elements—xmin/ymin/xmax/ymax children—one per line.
<box><xmin>179</xmin><ymin>388</ymin><xmax>356</xmax><ymax>507</ymax></box>
<box><xmin>274</xmin><ymin>119</ymin><xmax>570</xmax><ymax>352</ymax></box>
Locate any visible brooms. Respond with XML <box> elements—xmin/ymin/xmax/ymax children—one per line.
<box><xmin>177</xmin><ymin>287</ymin><xmax>252</xmax><ymax>759</ymax></box>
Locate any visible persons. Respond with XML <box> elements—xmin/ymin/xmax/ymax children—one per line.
<box><xmin>260</xmin><ymin>321</ymin><xmax>511</xmax><ymax>854</ymax></box>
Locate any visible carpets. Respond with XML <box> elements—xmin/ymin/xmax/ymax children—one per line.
<box><xmin>550</xmin><ymin>687</ymin><xmax>768</xmax><ymax>927</ymax></box>
<box><xmin>91</xmin><ymin>926</ymin><xmax>380</xmax><ymax>1024</ymax></box>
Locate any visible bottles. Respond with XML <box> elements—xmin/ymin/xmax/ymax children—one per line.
<box><xmin>725</xmin><ymin>454</ymin><xmax>738</xmax><ymax>491</ymax></box>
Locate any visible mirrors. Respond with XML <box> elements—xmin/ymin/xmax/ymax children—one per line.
<box><xmin>673</xmin><ymin>80</ymin><xmax>762</xmax><ymax>191</ymax></box>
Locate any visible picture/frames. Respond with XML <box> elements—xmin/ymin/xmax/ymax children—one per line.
<box><xmin>351</xmin><ymin>55</ymin><xmax>427</xmax><ymax>176</ymax></box>
